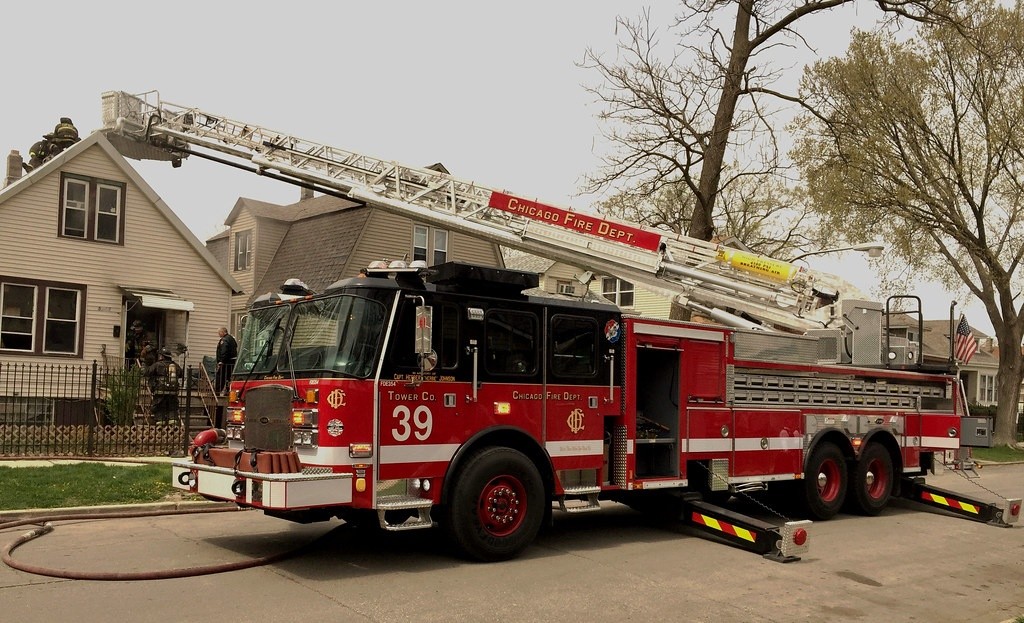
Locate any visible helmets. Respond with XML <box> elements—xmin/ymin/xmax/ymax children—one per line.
<box><xmin>131</xmin><ymin>320</ymin><xmax>145</xmax><ymax>330</ymax></box>
<box><xmin>157</xmin><ymin>348</ymin><xmax>172</xmax><ymax>358</ymax></box>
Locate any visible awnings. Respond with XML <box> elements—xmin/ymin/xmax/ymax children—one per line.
<box><xmin>139</xmin><ymin>294</ymin><xmax>195</xmax><ymax>312</ymax></box>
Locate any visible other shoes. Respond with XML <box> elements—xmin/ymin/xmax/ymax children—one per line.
<box><xmin>223</xmin><ymin>392</ymin><xmax>229</xmax><ymax>396</ymax></box>
<box><xmin>212</xmin><ymin>391</ymin><xmax>220</xmax><ymax>396</ymax></box>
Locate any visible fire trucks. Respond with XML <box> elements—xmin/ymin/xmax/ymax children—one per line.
<box><xmin>99</xmin><ymin>89</ymin><xmax>1023</xmax><ymax>564</ymax></box>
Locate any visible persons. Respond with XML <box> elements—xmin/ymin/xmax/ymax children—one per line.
<box><xmin>22</xmin><ymin>117</ymin><xmax>81</xmax><ymax>173</ymax></box>
<box><xmin>215</xmin><ymin>327</ymin><xmax>238</xmax><ymax>397</ymax></box>
<box><xmin>126</xmin><ymin>319</ymin><xmax>183</xmax><ymax>427</ymax></box>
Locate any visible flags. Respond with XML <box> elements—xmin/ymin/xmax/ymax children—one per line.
<box><xmin>955</xmin><ymin>314</ymin><xmax>977</xmax><ymax>366</ymax></box>
